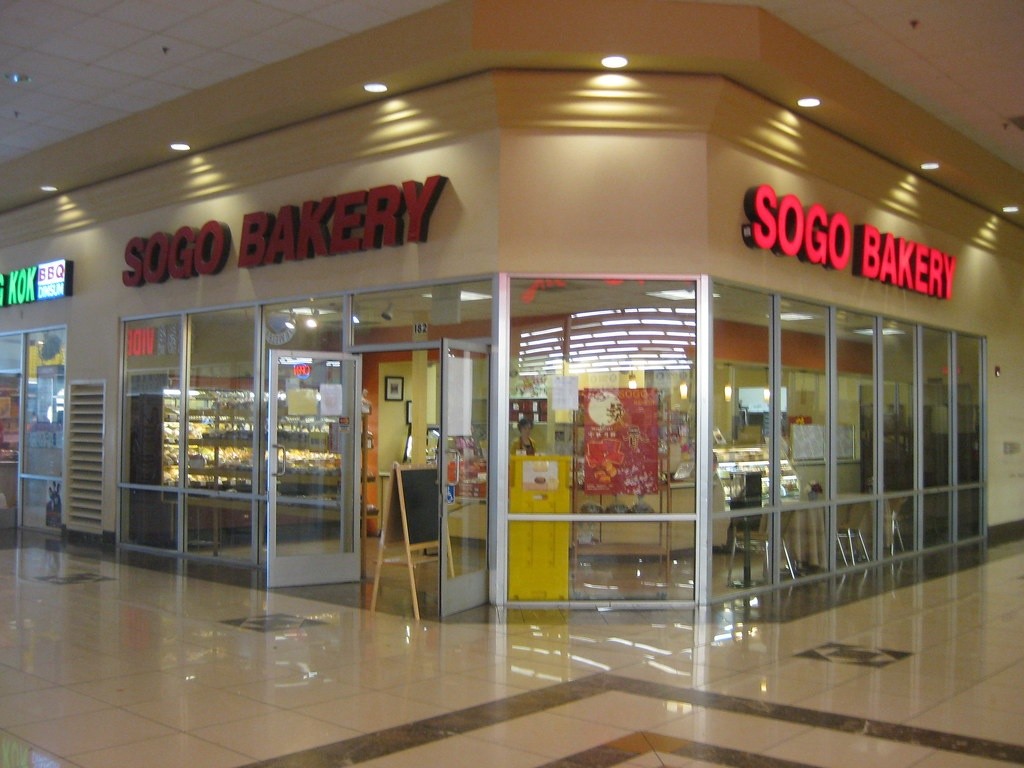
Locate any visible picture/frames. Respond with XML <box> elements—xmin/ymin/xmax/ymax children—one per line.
<box><xmin>406</xmin><ymin>401</ymin><xmax>412</xmax><ymax>425</ymax></box>
<box><xmin>384</xmin><ymin>376</ymin><xmax>404</xmax><ymax>401</ymax></box>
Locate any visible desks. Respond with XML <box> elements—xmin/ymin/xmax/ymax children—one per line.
<box><xmin>764</xmin><ymin>493</ymin><xmax>875</xmax><ymax>570</ymax></box>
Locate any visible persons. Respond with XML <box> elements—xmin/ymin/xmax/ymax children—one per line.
<box><xmin>510</xmin><ymin>419</ymin><xmax>538</xmax><ymax>456</ymax></box>
<box><xmin>451</xmin><ymin>436</ymin><xmax>478</xmax><ymax>457</ymax></box>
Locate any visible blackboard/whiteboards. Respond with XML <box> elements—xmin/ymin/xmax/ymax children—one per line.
<box><xmin>378</xmin><ymin>461</ymin><xmax>450</xmax><ymax>551</ymax></box>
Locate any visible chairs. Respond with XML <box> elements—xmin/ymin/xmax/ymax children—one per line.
<box><xmin>836</xmin><ymin>495</ymin><xmax>908</xmax><ymax>567</ymax></box>
<box><xmin>727</xmin><ymin>511</ymin><xmax>796</xmax><ymax>587</ymax></box>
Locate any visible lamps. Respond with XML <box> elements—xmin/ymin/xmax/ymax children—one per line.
<box><xmin>285</xmin><ymin>307</ymin><xmax>298</xmax><ymax>329</ymax></box>
<box><xmin>763</xmin><ymin>367</ymin><xmax>770</xmax><ymax>404</ymax></box>
<box><xmin>380</xmin><ymin>303</ymin><xmax>397</xmax><ymax>320</ymax></box>
<box><xmin>307</xmin><ymin>305</ymin><xmax>320</xmax><ymax>328</ymax></box>
<box><xmin>680</xmin><ymin>370</ymin><xmax>688</xmax><ymax>400</ymax></box>
<box><xmin>723</xmin><ymin>363</ymin><xmax>734</xmax><ymax>402</ymax></box>
<box><xmin>629</xmin><ymin>371</ymin><xmax>636</xmax><ymax>390</ymax></box>
